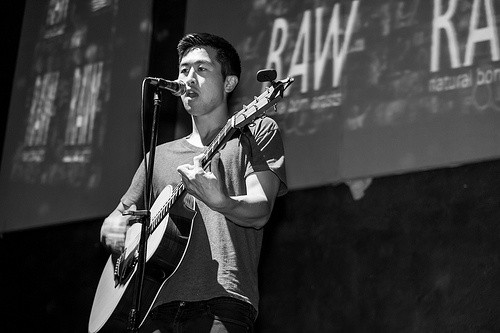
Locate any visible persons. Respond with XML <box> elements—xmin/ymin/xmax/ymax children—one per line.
<box><xmin>100</xmin><ymin>33</ymin><xmax>289</xmax><ymax>333</ymax></box>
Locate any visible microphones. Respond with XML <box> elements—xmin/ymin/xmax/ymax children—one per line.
<box><xmin>147</xmin><ymin>77</ymin><xmax>186</xmax><ymax>96</ymax></box>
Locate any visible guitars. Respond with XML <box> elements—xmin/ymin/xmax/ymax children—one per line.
<box><xmin>88</xmin><ymin>70</ymin><xmax>294</xmax><ymax>333</ymax></box>
